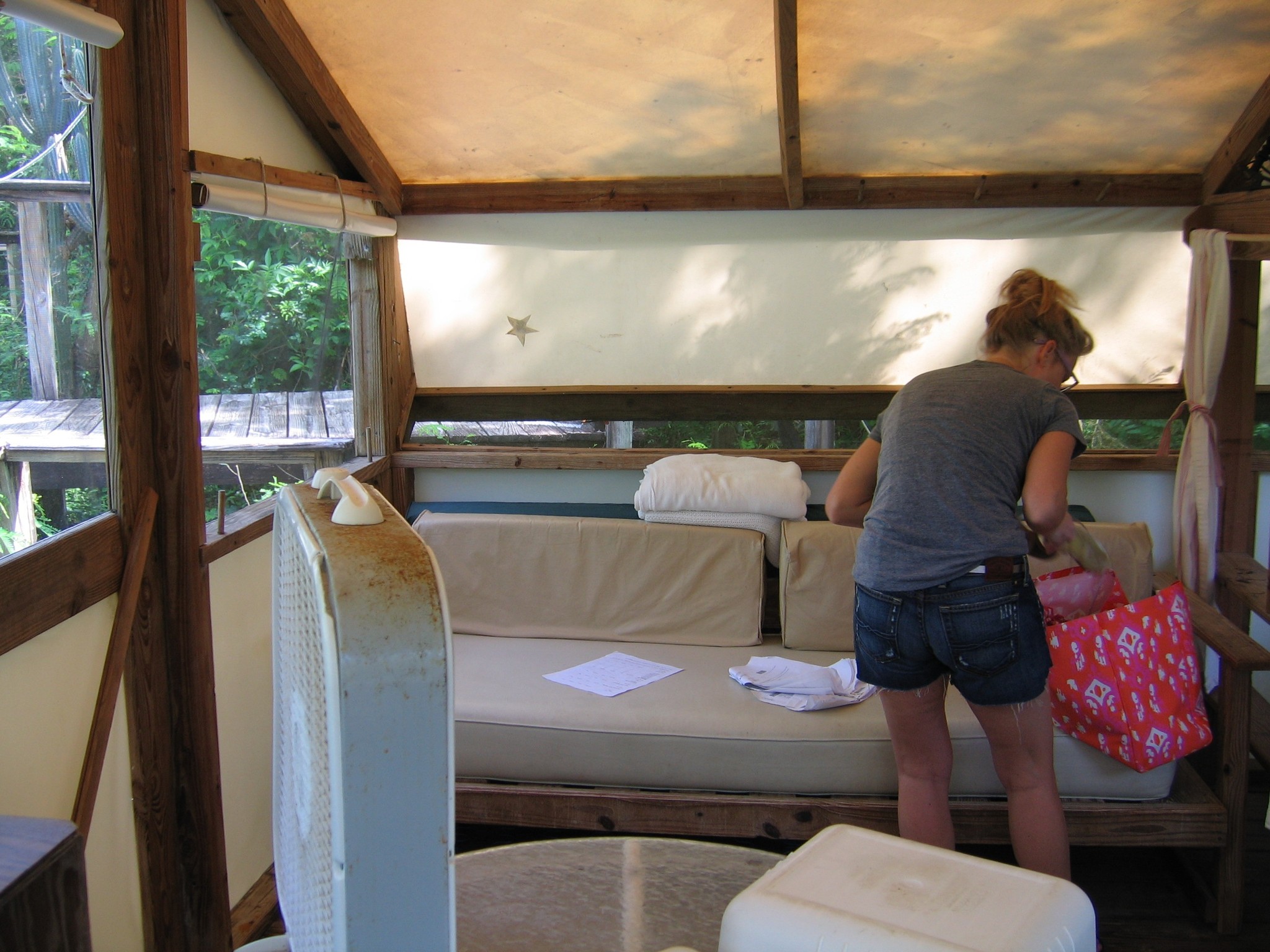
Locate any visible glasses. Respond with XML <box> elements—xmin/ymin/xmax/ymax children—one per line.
<box><xmin>1035</xmin><ymin>337</ymin><xmax>1081</xmax><ymax>392</ymax></box>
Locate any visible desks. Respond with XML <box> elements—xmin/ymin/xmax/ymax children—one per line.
<box><xmin>454</xmin><ymin>836</ymin><xmax>787</xmax><ymax>952</ymax></box>
<box><xmin>0</xmin><ymin>815</ymin><xmax>92</xmax><ymax>952</ymax></box>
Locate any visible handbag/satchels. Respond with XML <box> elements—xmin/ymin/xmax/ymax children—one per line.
<box><xmin>1033</xmin><ymin>564</ymin><xmax>1214</xmax><ymax>773</ymax></box>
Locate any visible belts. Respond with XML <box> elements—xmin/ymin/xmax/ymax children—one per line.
<box><xmin>969</xmin><ymin>566</ymin><xmax>1022</xmax><ymax>574</ymax></box>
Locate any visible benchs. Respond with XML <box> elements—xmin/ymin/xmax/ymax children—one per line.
<box><xmin>410</xmin><ymin>508</ymin><xmax>1270</xmax><ymax>934</ymax></box>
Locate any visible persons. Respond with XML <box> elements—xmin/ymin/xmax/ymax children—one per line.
<box><xmin>826</xmin><ymin>269</ymin><xmax>1102</xmax><ymax>952</ymax></box>
